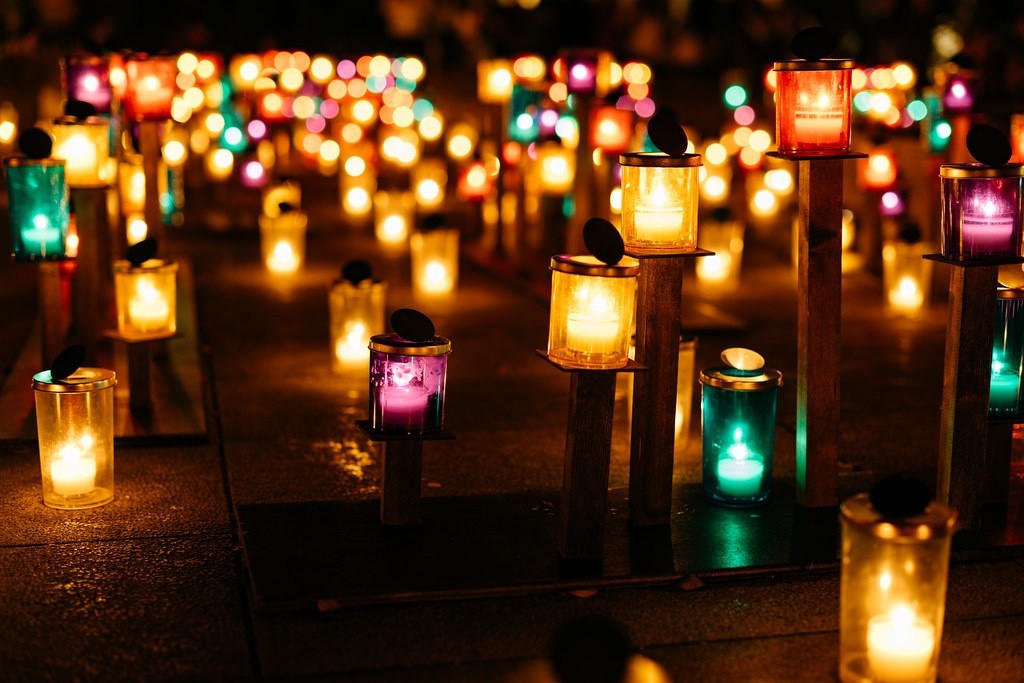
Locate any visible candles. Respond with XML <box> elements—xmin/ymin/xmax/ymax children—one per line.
<box><xmin>21</xmin><ymin>218</ymin><xmax>60</xmax><ymax>255</ymax></box>
<box><xmin>129</xmin><ymin>279</ymin><xmax>168</xmax><ymax>330</ymax></box>
<box><xmin>963</xmin><ymin>198</ymin><xmax>1013</xmax><ymax>253</ymax></box>
<box><xmin>866</xmin><ymin>604</ymin><xmax>935</xmax><ymax>683</ymax></box>
<box><xmin>793</xmin><ymin>87</ymin><xmax>845</xmax><ymax>143</ymax></box>
<box><xmin>48</xmin><ymin>438</ymin><xmax>95</xmax><ymax>497</ymax></box>
<box><xmin>990</xmin><ymin>361</ymin><xmax>1019</xmax><ymax>409</ymax></box>
<box><xmin>379</xmin><ymin>369</ymin><xmax>430</xmax><ymax>427</ymax></box>
<box><xmin>633</xmin><ymin>184</ymin><xmax>683</xmax><ymax>242</ymax></box>
<box><xmin>566</xmin><ymin>288</ymin><xmax>621</xmax><ymax>355</ymax></box>
<box><xmin>715</xmin><ymin>442</ymin><xmax>764</xmax><ymax>496</ymax></box>
